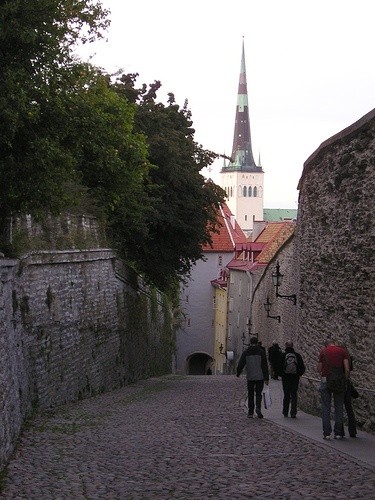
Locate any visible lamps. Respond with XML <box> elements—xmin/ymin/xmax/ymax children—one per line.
<box><xmin>240</xmin><ymin>331</ymin><xmax>250</xmax><ymax>347</ymax></box>
<box><xmin>269</xmin><ymin>261</ymin><xmax>298</xmax><ymax>306</ymax></box>
<box><xmin>218</xmin><ymin>343</ymin><xmax>226</xmax><ymax>355</ymax></box>
<box><xmin>244</xmin><ymin>314</ymin><xmax>259</xmax><ymax>338</ymax></box>
<box><xmin>264</xmin><ymin>293</ymin><xmax>281</xmax><ymax>323</ymax></box>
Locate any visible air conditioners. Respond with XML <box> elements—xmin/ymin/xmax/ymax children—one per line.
<box><xmin>226</xmin><ymin>350</ymin><xmax>234</xmax><ymax>360</ymax></box>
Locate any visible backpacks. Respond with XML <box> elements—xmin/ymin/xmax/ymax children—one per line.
<box><xmin>284</xmin><ymin>350</ymin><xmax>297</xmax><ymax>374</ymax></box>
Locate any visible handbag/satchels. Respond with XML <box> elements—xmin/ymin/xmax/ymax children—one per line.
<box><xmin>262</xmin><ymin>384</ymin><xmax>272</xmax><ymax>409</ymax></box>
<box><xmin>348</xmin><ymin>382</ymin><xmax>359</xmax><ymax>399</ymax></box>
<box><xmin>326</xmin><ymin>365</ymin><xmax>347</xmax><ymax>394</ymax></box>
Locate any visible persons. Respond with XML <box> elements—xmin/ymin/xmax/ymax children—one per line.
<box><xmin>258</xmin><ymin>339</ymin><xmax>280</xmax><ymax>381</ymax></box>
<box><xmin>317</xmin><ymin>338</ymin><xmax>349</xmax><ymax>440</ymax></box>
<box><xmin>338</xmin><ymin>342</ymin><xmax>359</xmax><ymax>438</ymax></box>
<box><xmin>277</xmin><ymin>340</ymin><xmax>305</xmax><ymax>418</ymax></box>
<box><xmin>236</xmin><ymin>337</ymin><xmax>269</xmax><ymax>419</ymax></box>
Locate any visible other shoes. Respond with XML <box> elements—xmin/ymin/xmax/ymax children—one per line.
<box><xmin>334</xmin><ymin>435</ymin><xmax>341</xmax><ymax>440</ymax></box>
<box><xmin>255</xmin><ymin>408</ymin><xmax>263</xmax><ymax>419</ymax></box>
<box><xmin>284</xmin><ymin>414</ymin><xmax>288</xmax><ymax>417</ymax></box>
<box><xmin>291</xmin><ymin>415</ymin><xmax>296</xmax><ymax>418</ymax></box>
<box><xmin>351</xmin><ymin>435</ymin><xmax>356</xmax><ymax>438</ymax></box>
<box><xmin>248</xmin><ymin>414</ymin><xmax>253</xmax><ymax>418</ymax></box>
<box><xmin>323</xmin><ymin>436</ymin><xmax>330</xmax><ymax>440</ymax></box>
